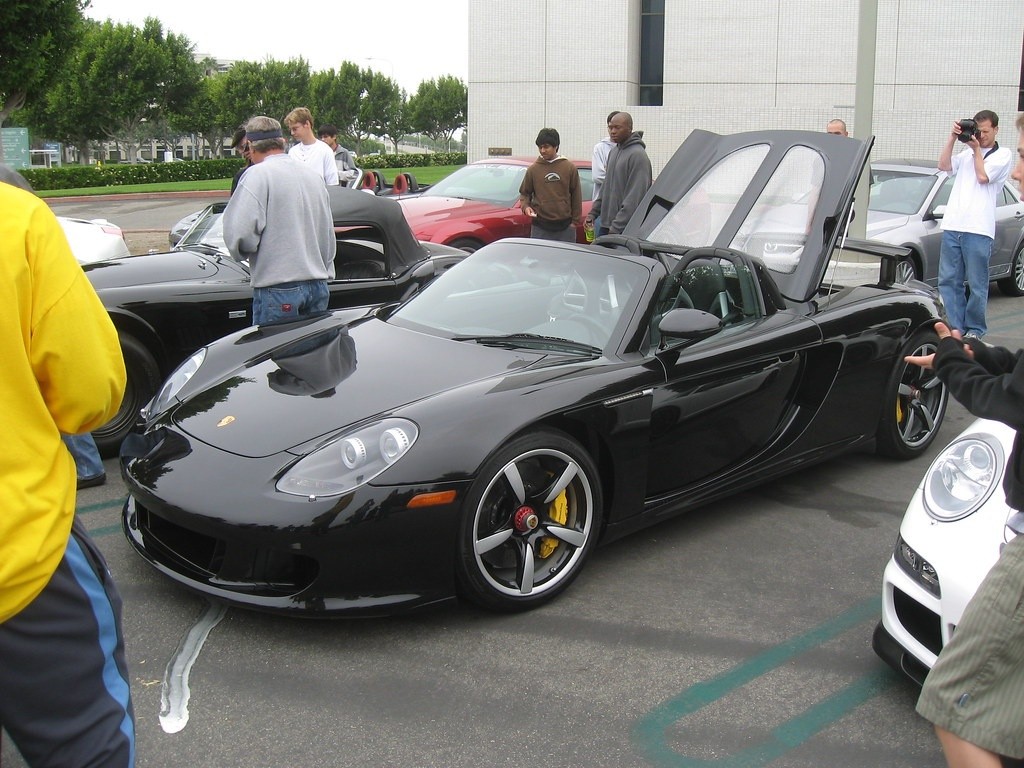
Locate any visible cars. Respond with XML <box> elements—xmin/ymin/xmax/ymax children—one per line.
<box><xmin>56</xmin><ymin>217</ymin><xmax>131</xmax><ymax>266</ymax></box>
<box><xmin>719</xmin><ymin>156</ymin><xmax>1024</xmax><ymax>298</ymax></box>
<box><xmin>121</xmin><ymin>157</ymin><xmax>152</xmax><ymax>164</ymax></box>
<box><xmin>871</xmin><ymin>415</ymin><xmax>1024</xmax><ymax>689</ymax></box>
<box><xmin>161</xmin><ymin>158</ymin><xmax>183</xmax><ymax>162</ymax></box>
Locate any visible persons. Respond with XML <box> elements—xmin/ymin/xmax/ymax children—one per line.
<box><xmin>583</xmin><ymin>112</ymin><xmax>652</xmax><ymax>249</ymax></box>
<box><xmin>805</xmin><ymin>119</ymin><xmax>875</xmax><ymax>238</ymax></box>
<box><xmin>905</xmin><ymin>113</ymin><xmax>1024</xmax><ymax>768</ymax></box>
<box><xmin>938</xmin><ymin>110</ymin><xmax>1012</xmax><ymax>340</ymax></box>
<box><xmin>223</xmin><ymin>116</ymin><xmax>336</xmax><ymax>325</ymax></box>
<box><xmin>592</xmin><ymin>111</ymin><xmax>622</xmax><ymax>238</ymax></box>
<box><xmin>230</xmin><ymin>123</ymin><xmax>256</xmax><ymax>198</ymax></box>
<box><xmin>318</xmin><ymin>123</ymin><xmax>359</xmax><ymax>188</ymax></box>
<box><xmin>0</xmin><ymin>147</ymin><xmax>135</xmax><ymax>768</ymax></box>
<box><xmin>519</xmin><ymin>128</ymin><xmax>582</xmax><ymax>277</ymax></box>
<box><xmin>284</xmin><ymin>107</ymin><xmax>339</xmax><ymax>186</ymax></box>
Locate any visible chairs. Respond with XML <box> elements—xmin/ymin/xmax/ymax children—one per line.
<box><xmin>361</xmin><ymin>171</ymin><xmax>376</xmax><ymax>190</ymax></box>
<box><xmin>543</xmin><ymin>236</ymin><xmax>641</xmax><ymax>330</ymax></box>
<box><xmin>392</xmin><ymin>173</ymin><xmax>408</xmax><ymax>195</ymax></box>
<box><xmin>639</xmin><ymin>246</ymin><xmax>760</xmax><ymax>350</ymax></box>
<box><xmin>336</xmin><ymin>259</ymin><xmax>385</xmax><ymax>279</ymax></box>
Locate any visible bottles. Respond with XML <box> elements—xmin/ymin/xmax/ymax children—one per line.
<box><xmin>585</xmin><ymin>219</ymin><xmax>596</xmax><ymax>242</ymax></box>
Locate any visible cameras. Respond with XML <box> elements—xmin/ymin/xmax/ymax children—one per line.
<box><xmin>958</xmin><ymin>118</ymin><xmax>980</xmax><ymax>143</ymax></box>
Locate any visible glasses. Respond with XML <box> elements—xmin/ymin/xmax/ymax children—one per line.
<box><xmin>237</xmin><ymin>143</ymin><xmax>246</xmax><ymax>153</ymax></box>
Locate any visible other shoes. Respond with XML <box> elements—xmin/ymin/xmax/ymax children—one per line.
<box><xmin>964</xmin><ymin>333</ymin><xmax>982</xmax><ymax>341</ymax></box>
<box><xmin>75</xmin><ymin>473</ymin><xmax>106</xmax><ymax>490</ymax></box>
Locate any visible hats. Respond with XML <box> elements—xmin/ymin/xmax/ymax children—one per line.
<box><xmin>231</xmin><ymin>124</ymin><xmax>246</xmax><ymax>149</ymax></box>
<box><xmin>243</xmin><ymin>130</ymin><xmax>283</xmax><ymax>152</ymax></box>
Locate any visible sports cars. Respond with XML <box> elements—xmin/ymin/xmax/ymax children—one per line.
<box><xmin>335</xmin><ymin>156</ymin><xmax>712</xmax><ymax>256</ymax></box>
<box><xmin>118</xmin><ymin>128</ymin><xmax>956</xmax><ymax>621</ymax></box>
<box><xmin>80</xmin><ymin>186</ymin><xmax>475</xmax><ymax>459</ymax></box>
<box><xmin>169</xmin><ymin>168</ymin><xmax>433</xmax><ymax>251</ymax></box>
